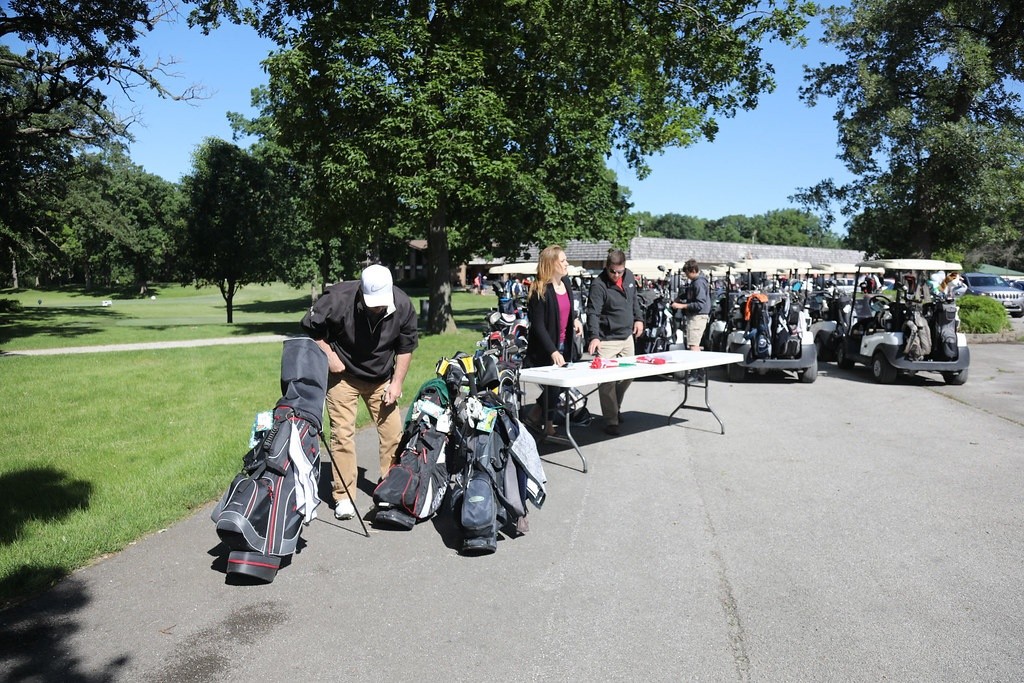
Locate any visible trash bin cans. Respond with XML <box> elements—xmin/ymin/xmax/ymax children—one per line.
<box><xmin>419</xmin><ymin>299</ymin><xmax>430</xmax><ymax>318</ymax></box>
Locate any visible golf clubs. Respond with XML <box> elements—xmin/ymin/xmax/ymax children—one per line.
<box><xmin>432</xmin><ymin>306</ymin><xmax>530</xmax><ymax>417</ymax></box>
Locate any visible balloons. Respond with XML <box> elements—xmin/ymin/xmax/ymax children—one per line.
<box><xmin>925</xmin><ymin>271</ymin><xmax>968</xmax><ymax>304</ymax></box>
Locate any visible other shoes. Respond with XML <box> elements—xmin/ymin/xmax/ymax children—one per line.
<box><xmin>607</xmin><ymin>425</ymin><xmax>622</xmax><ymax>434</ymax></box>
<box><xmin>522</xmin><ymin>417</ymin><xmax>543</xmax><ymax>432</ymax></box>
<box><xmin>618</xmin><ymin>411</ymin><xmax>624</xmax><ymax>422</ymax></box>
<box><xmin>681</xmin><ymin>370</ymin><xmax>706</xmax><ymax>385</ymax></box>
<box><xmin>552</xmin><ymin>432</ymin><xmax>568</xmax><ymax>439</ymax></box>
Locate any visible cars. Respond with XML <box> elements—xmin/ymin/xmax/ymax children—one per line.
<box><xmin>835</xmin><ymin>278</ymin><xmax>861</xmax><ymax>292</ymax></box>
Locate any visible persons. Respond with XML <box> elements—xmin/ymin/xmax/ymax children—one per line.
<box><xmin>725</xmin><ymin>270</ymin><xmax>883</xmax><ymax>293</ymax></box>
<box><xmin>512</xmin><ymin>276</ymin><xmax>535</xmax><ymax>301</ymax></box>
<box><xmin>670</xmin><ymin>259</ymin><xmax>711</xmax><ymax>383</ymax></box>
<box><xmin>589</xmin><ymin>250</ymin><xmax>645</xmax><ymax>437</ymax></box>
<box><xmin>301</xmin><ymin>264</ymin><xmax>419</xmax><ymax>521</ymax></box>
<box><xmin>473</xmin><ymin>272</ymin><xmax>486</xmax><ymax>297</ymax></box>
<box><xmin>522</xmin><ymin>245</ymin><xmax>585</xmax><ymax>442</ymax></box>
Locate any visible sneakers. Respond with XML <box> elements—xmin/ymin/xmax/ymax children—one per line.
<box><xmin>334</xmin><ymin>499</ymin><xmax>356</xmax><ymax>520</ymax></box>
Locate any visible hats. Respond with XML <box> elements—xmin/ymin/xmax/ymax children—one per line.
<box><xmin>361</xmin><ymin>265</ymin><xmax>393</xmax><ymax>308</ymax></box>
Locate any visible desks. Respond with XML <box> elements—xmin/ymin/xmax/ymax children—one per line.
<box><xmin>515</xmin><ymin>350</ymin><xmax>744</xmax><ymax>473</ymax></box>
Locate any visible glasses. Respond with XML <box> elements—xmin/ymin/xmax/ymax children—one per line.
<box><xmin>609</xmin><ymin>269</ymin><xmax>623</xmax><ymax>274</ymax></box>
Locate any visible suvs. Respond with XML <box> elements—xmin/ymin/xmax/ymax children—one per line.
<box><xmin>960</xmin><ymin>272</ymin><xmax>1024</xmax><ymax>318</ymax></box>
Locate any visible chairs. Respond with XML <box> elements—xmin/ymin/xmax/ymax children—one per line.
<box><xmin>885</xmin><ymin>302</ymin><xmax>907</xmax><ymax>333</ymax></box>
